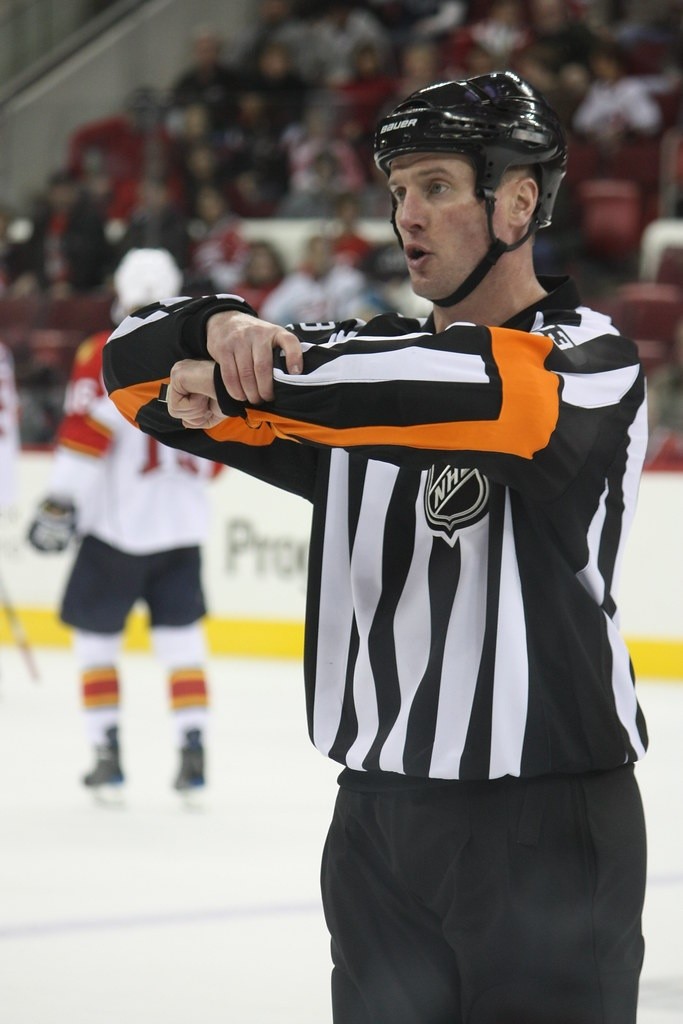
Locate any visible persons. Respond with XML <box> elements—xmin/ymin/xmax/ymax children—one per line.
<box><xmin>102</xmin><ymin>75</ymin><xmax>649</xmax><ymax>1024</ymax></box>
<box><xmin>0</xmin><ymin>0</ymin><xmax>683</xmax><ymax>443</ymax></box>
<box><xmin>26</xmin><ymin>246</ymin><xmax>229</xmax><ymax>789</ymax></box>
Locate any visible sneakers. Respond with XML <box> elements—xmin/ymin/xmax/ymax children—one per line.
<box><xmin>82</xmin><ymin>725</ymin><xmax>127</xmax><ymax>811</ymax></box>
<box><xmin>170</xmin><ymin>728</ymin><xmax>204</xmax><ymax>812</ymax></box>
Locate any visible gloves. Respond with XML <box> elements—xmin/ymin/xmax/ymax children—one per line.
<box><xmin>26</xmin><ymin>495</ymin><xmax>79</xmax><ymax>554</ymax></box>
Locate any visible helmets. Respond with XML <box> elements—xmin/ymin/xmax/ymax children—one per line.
<box><xmin>109</xmin><ymin>248</ymin><xmax>180</xmax><ymax>319</ymax></box>
<box><xmin>371</xmin><ymin>71</ymin><xmax>569</xmax><ymax>229</ymax></box>
